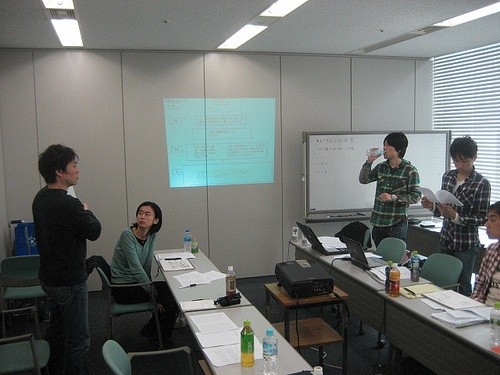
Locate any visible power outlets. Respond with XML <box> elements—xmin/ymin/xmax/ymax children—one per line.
<box><xmin>293</xmin><ymin>227</ymin><xmax>298</xmax><ymax>236</ymax></box>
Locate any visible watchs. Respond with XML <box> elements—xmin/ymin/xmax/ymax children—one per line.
<box><xmin>391</xmin><ymin>194</ymin><xmax>397</xmax><ymax>202</ymax></box>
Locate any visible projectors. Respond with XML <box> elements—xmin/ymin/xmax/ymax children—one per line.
<box><xmin>275</xmin><ymin>258</ymin><xmax>335</xmax><ymax>298</ymax></box>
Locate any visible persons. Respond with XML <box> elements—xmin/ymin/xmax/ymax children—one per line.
<box><xmin>359</xmin><ymin>132</ymin><xmax>422</xmax><ymax>247</ymax></box>
<box><xmin>420</xmin><ymin>137</ymin><xmax>490</xmax><ymax>298</ymax></box>
<box><xmin>111</xmin><ymin>201</ymin><xmax>180</xmax><ymax>347</ymax></box>
<box><xmin>469</xmin><ymin>201</ymin><xmax>500</xmax><ymax>308</ymax></box>
<box><xmin>32</xmin><ymin>144</ymin><xmax>101</xmax><ymax>375</ymax></box>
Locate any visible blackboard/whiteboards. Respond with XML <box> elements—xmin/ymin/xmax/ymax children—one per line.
<box><xmin>300</xmin><ymin>131</ymin><xmax>451</xmax><ymax>223</ymax></box>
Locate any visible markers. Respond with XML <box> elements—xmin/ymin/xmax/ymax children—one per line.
<box><xmin>327</xmin><ymin>215</ymin><xmax>337</xmax><ymax>218</ymax></box>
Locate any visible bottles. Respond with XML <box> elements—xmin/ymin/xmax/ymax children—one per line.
<box><xmin>225</xmin><ymin>266</ymin><xmax>236</xmax><ymax>296</ymax></box>
<box><xmin>490</xmin><ymin>301</ymin><xmax>500</xmax><ymax>346</ymax></box>
<box><xmin>385</xmin><ymin>261</ymin><xmax>393</xmax><ymax>293</ymax></box>
<box><xmin>191</xmin><ymin>237</ymin><xmax>199</xmax><ymax>254</ymax></box>
<box><xmin>411</xmin><ymin>250</ymin><xmax>420</xmax><ymax>282</ymax></box>
<box><xmin>240</xmin><ymin>319</ymin><xmax>254</xmax><ymax>367</ymax></box>
<box><xmin>184</xmin><ymin>230</ymin><xmax>192</xmax><ymax>253</ymax></box>
<box><xmin>262</xmin><ymin>328</ymin><xmax>278</xmax><ymax>375</ymax></box>
<box><xmin>363</xmin><ymin>148</ymin><xmax>386</xmax><ymax>157</ymax></box>
<box><xmin>389</xmin><ymin>263</ymin><xmax>400</xmax><ymax>298</ymax></box>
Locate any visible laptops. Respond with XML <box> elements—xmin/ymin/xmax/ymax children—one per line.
<box><xmin>295</xmin><ymin>220</ymin><xmax>349</xmax><ymax>256</ymax></box>
<box><xmin>342</xmin><ymin>237</ymin><xmax>388</xmax><ymax>270</ymax></box>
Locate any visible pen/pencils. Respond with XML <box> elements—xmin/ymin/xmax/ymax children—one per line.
<box><xmin>192</xmin><ymin>299</ymin><xmax>205</xmax><ymax>301</ymax></box>
<box><xmin>404</xmin><ymin>288</ymin><xmax>415</xmax><ymax>295</ymax></box>
<box><xmin>179</xmin><ymin>284</ymin><xmax>196</xmax><ymax>289</ymax></box>
<box><xmin>165</xmin><ymin>258</ymin><xmax>181</xmax><ymax>260</ymax></box>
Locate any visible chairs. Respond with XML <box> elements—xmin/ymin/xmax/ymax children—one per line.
<box><xmin>0</xmin><ymin>221</ymin><xmax>464</xmax><ymax>375</ymax></box>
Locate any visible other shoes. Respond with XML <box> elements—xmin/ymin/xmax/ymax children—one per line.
<box><xmin>152</xmin><ymin>336</ymin><xmax>178</xmax><ymax>349</ymax></box>
<box><xmin>140</xmin><ymin>324</ymin><xmax>157</xmax><ymax>338</ymax></box>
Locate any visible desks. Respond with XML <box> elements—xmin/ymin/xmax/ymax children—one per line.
<box><xmin>153</xmin><ymin>248</ymin><xmax>250</xmax><ymax>313</ymax></box>
<box><xmin>377</xmin><ymin>284</ymin><xmax>500</xmax><ymax>375</ymax></box>
<box><xmin>407</xmin><ymin>216</ymin><xmax>498</xmax><ymax>291</ymax></box>
<box><xmin>288</xmin><ymin>236</ymin><xmax>433</xmax><ymax>331</ymax></box>
<box><xmin>184</xmin><ymin>307</ymin><xmax>315</xmax><ymax>375</ymax></box>
